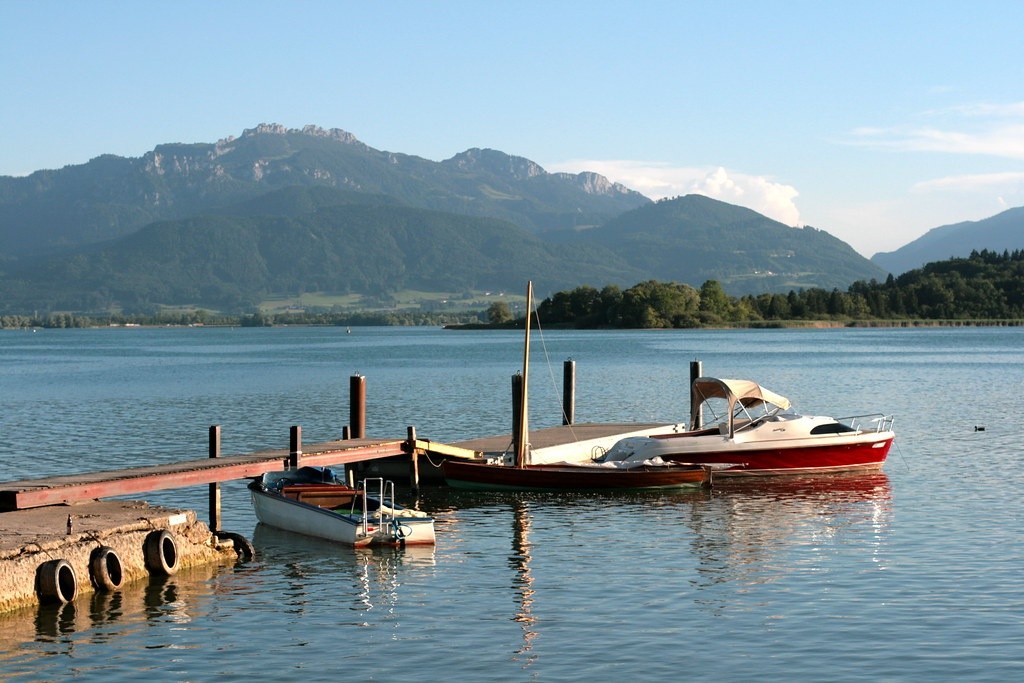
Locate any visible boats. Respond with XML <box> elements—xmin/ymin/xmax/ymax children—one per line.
<box><xmin>591</xmin><ymin>376</ymin><xmax>897</xmax><ymax>479</ymax></box>
<box><xmin>248</xmin><ymin>466</ymin><xmax>437</xmax><ymax>550</ymax></box>
<box><xmin>439</xmin><ymin>457</ymin><xmax>708</xmax><ymax>492</ymax></box>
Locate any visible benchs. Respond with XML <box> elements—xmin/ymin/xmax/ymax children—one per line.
<box><xmin>281</xmin><ymin>485</ymin><xmax>363</xmax><ymax>505</ymax></box>
